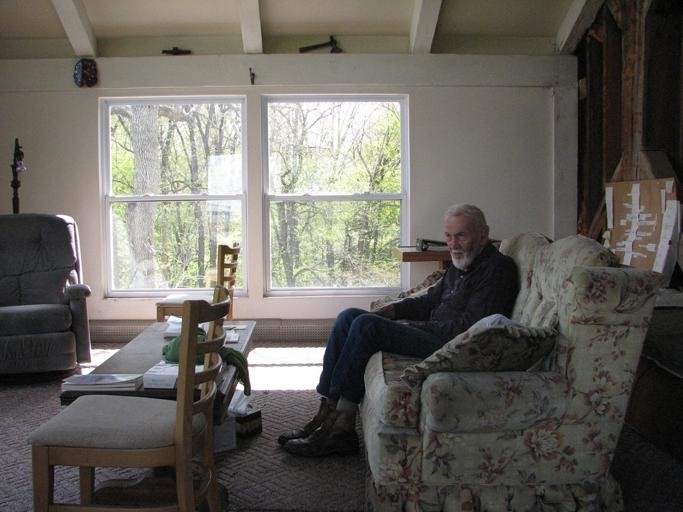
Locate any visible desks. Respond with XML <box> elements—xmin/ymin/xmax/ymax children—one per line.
<box><xmin>60</xmin><ymin>321</ymin><xmax>258</xmax><ymax>424</ymax></box>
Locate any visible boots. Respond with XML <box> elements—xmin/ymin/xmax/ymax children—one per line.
<box><xmin>278</xmin><ymin>397</ymin><xmax>360</xmax><ymax>456</ymax></box>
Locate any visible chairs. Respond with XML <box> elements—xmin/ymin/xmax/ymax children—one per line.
<box><xmin>26</xmin><ymin>284</ymin><xmax>231</xmax><ymax>512</ymax></box>
<box><xmin>156</xmin><ymin>243</ymin><xmax>240</xmax><ymax>321</ymax></box>
<box><xmin>0</xmin><ymin>213</ymin><xmax>92</xmax><ymax>380</ymax></box>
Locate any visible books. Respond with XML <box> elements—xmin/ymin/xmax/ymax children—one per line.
<box><xmin>61</xmin><ymin>362</ymin><xmax>204</xmax><ymax>392</ymax></box>
<box><xmin>164</xmin><ymin>316</ymin><xmax>208</xmax><ymax>337</ymax></box>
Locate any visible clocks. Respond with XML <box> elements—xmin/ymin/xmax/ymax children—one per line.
<box><xmin>74</xmin><ymin>59</ymin><xmax>98</xmax><ymax>86</ymax></box>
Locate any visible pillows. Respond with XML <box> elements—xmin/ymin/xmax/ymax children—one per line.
<box><xmin>401</xmin><ymin>313</ymin><xmax>560</xmax><ymax>391</ymax></box>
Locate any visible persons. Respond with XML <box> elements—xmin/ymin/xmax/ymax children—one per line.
<box><xmin>278</xmin><ymin>203</ymin><xmax>517</xmax><ymax>457</ymax></box>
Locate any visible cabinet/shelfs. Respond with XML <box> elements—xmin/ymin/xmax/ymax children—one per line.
<box><xmin>393</xmin><ymin>245</ymin><xmax>450</xmax><ymax>270</ymax></box>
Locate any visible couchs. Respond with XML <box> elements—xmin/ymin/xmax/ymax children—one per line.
<box><xmin>359</xmin><ymin>231</ymin><xmax>665</xmax><ymax>512</ymax></box>
